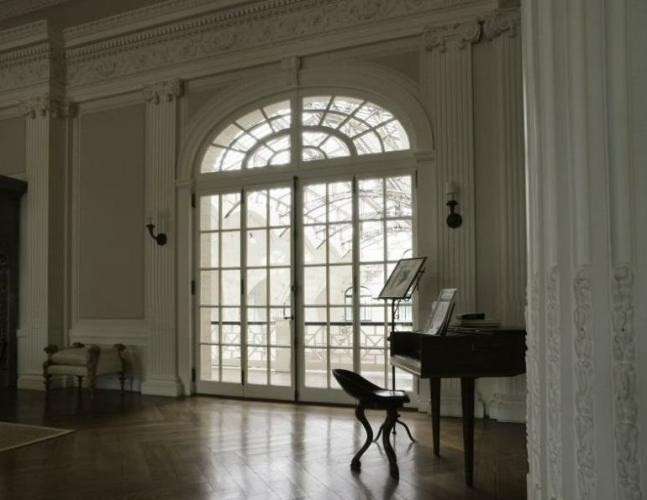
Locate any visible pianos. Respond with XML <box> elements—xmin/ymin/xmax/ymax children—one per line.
<box><xmin>389</xmin><ymin>327</ymin><xmax>526</xmax><ymax>490</ymax></box>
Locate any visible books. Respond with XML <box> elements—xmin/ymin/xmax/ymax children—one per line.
<box><xmin>447</xmin><ymin>313</ymin><xmax>505</xmax><ymax>334</ymax></box>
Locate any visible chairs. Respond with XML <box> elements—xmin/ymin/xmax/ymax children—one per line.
<box><xmin>332</xmin><ymin>369</ymin><xmax>410</xmax><ymax>478</ymax></box>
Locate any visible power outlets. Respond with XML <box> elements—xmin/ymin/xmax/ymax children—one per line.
<box><xmin>16</xmin><ymin>329</ymin><xmax>26</xmax><ymax>338</ymax></box>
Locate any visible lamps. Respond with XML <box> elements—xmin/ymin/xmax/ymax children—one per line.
<box><xmin>445</xmin><ymin>181</ymin><xmax>462</xmax><ymax>228</ymax></box>
<box><xmin>145</xmin><ymin>212</ymin><xmax>167</xmax><ymax>246</ymax></box>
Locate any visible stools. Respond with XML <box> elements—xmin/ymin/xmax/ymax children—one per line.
<box><xmin>43</xmin><ymin>342</ymin><xmax>126</xmax><ymax>400</ymax></box>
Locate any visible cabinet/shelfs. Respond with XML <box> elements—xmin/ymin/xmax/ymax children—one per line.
<box><xmin>0</xmin><ymin>174</ymin><xmax>28</xmax><ymax>389</ymax></box>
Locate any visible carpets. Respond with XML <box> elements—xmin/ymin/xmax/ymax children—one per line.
<box><xmin>0</xmin><ymin>421</ymin><xmax>79</xmax><ymax>452</ymax></box>
